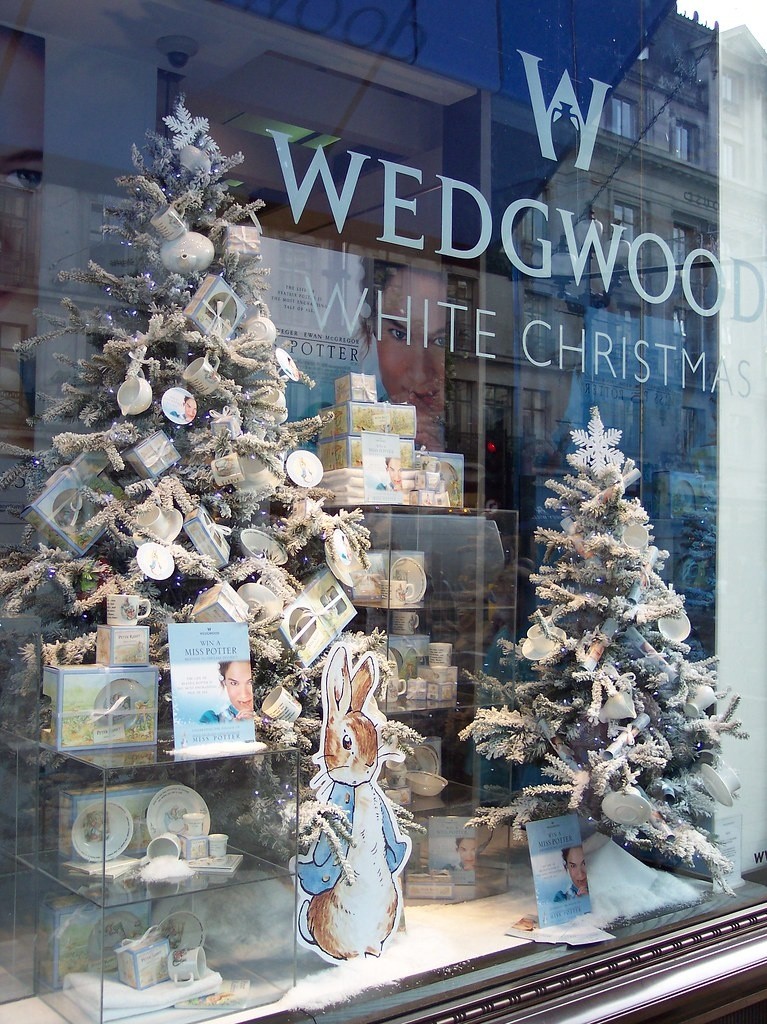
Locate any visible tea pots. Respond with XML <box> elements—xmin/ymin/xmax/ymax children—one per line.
<box><xmin>160</xmin><ymin>226</ymin><xmax>215</xmax><ymax>273</ymax></box>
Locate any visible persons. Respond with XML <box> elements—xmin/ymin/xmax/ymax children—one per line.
<box><xmin>304</xmin><ymin>258</ymin><xmax>446</xmax><ymax>448</ymax></box>
<box><xmin>377</xmin><ymin>457</ymin><xmax>401</xmax><ymax>491</ymax></box>
<box><xmin>200</xmin><ymin>661</ymin><xmax>253</xmax><ymax>724</ymax></box>
<box><xmin>554</xmin><ymin>846</ymin><xmax>588</xmax><ymax>899</ymax></box>
<box><xmin>438</xmin><ymin>838</ymin><xmax>475</xmax><ymax>870</ymax></box>
<box><xmin>170</xmin><ymin>398</ymin><xmax>197</xmax><ymax>420</ymax></box>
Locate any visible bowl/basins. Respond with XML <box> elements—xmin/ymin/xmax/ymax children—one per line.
<box><xmin>404</xmin><ymin>746</ymin><xmax>440</xmax><ymax>776</ymax></box>
<box><xmin>244</xmin><ymin>317</ymin><xmax>276</xmax><ymax>346</ymax></box>
<box><xmin>240</xmin><ymin>529</ymin><xmax>288</xmax><ymax>565</ymax></box>
<box><xmin>658</xmin><ymin>609</ymin><xmax>692</xmax><ymax>643</ymax></box>
<box><xmin>406</xmin><ymin>771</ymin><xmax>448</xmax><ymax>797</ymax></box>
<box><xmin>71</xmin><ymin>800</ymin><xmax>134</xmax><ymax>864</ymax></box>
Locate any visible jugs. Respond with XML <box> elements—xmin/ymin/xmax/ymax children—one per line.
<box><xmin>116</xmin><ymin>367</ymin><xmax>153</xmax><ymax>416</ymax></box>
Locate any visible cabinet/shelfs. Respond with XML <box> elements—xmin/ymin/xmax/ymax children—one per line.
<box><xmin>334</xmin><ymin>503</ymin><xmax>524</xmax><ymax>909</ymax></box>
<box><xmin>10</xmin><ymin>731</ymin><xmax>300</xmax><ymax>1024</ymax></box>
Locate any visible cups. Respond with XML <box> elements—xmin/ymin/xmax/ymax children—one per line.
<box><xmin>261</xmin><ymin>686</ymin><xmax>303</xmax><ymax>723</ymax></box>
<box><xmin>167</xmin><ymin>946</ymin><xmax>206</xmax><ymax>987</ymax></box>
<box><xmin>136</xmin><ymin>506</ymin><xmax>170</xmax><ymax>539</ymax></box>
<box><xmin>210</xmin><ymin>447</ymin><xmax>247</xmax><ymax>486</ymax></box>
<box><xmin>148</xmin><ymin>202</ymin><xmax>186</xmax><ymax>241</ymax></box>
<box><xmin>183</xmin><ymin>813</ymin><xmax>204</xmax><ymax>837</ymax></box>
<box><xmin>560</xmin><ymin>516</ymin><xmax>576</xmax><ymax>533</ymax></box>
<box><xmin>147</xmin><ymin>832</ymin><xmax>182</xmax><ymax>864</ymax></box>
<box><xmin>427</xmin><ymin>643</ymin><xmax>453</xmax><ymax>668</ymax></box>
<box><xmin>376</xmin><ymin>676</ymin><xmax>407</xmax><ymax>703</ymax></box>
<box><xmin>619</xmin><ymin>524</ymin><xmax>648</xmax><ymax>552</ymax></box>
<box><xmin>683</xmin><ymin>683</ymin><xmax>718</xmax><ymax>718</ymax></box>
<box><xmin>181</xmin><ymin>145</ymin><xmax>211</xmax><ymax>174</ymax></box>
<box><xmin>380</xmin><ymin>580</ymin><xmax>415</xmax><ymax>607</ymax></box>
<box><xmin>392</xmin><ymin>611</ymin><xmax>419</xmax><ymax>636</ymax></box>
<box><xmin>526</xmin><ymin>618</ymin><xmax>555</xmax><ymax>654</ymax></box>
<box><xmin>718</xmin><ymin>764</ymin><xmax>742</xmax><ymax>794</ymax></box>
<box><xmin>599</xmin><ymin>690</ymin><xmax>636</xmax><ymax>724</ymax></box>
<box><xmin>208</xmin><ymin>833</ymin><xmax>229</xmax><ymax>857</ymax></box>
<box><xmin>617</xmin><ymin>784</ymin><xmax>641</xmax><ymax>797</ymax></box>
<box><xmin>422</xmin><ymin>457</ymin><xmax>441</xmax><ymax>473</ymax></box>
<box><xmin>182</xmin><ymin>353</ymin><xmax>222</xmax><ymax>396</ymax></box>
<box><xmin>106</xmin><ymin>593</ymin><xmax>152</xmax><ymax>626</ymax></box>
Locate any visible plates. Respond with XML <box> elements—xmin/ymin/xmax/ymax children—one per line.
<box><xmin>133</xmin><ymin>507</ymin><xmax>183</xmax><ymax>549</ymax></box>
<box><xmin>155</xmin><ymin>911</ymin><xmax>206</xmax><ymax>951</ymax></box>
<box><xmin>146</xmin><ymin>785</ymin><xmax>211</xmax><ymax>851</ymax></box>
<box><xmin>521</xmin><ymin>627</ymin><xmax>567</xmax><ymax>661</ymax></box>
<box><xmin>286</xmin><ymin>449</ymin><xmax>324</xmax><ymax>489</ymax></box>
<box><xmin>238</xmin><ymin>582</ymin><xmax>282</xmax><ymax>631</ymax></box>
<box><xmin>388</xmin><ymin>557</ymin><xmax>427</xmax><ymax>604</ymax></box>
<box><xmin>699</xmin><ymin>763</ymin><xmax>734</xmax><ymax>807</ymax></box>
<box><xmin>601</xmin><ymin>791</ymin><xmax>651</xmax><ymax>826</ymax></box>
<box><xmin>136</xmin><ymin>542</ymin><xmax>175</xmax><ymax>580</ymax></box>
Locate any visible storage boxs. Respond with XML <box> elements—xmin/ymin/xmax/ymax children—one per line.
<box><xmin>180</xmin><ymin>226</ymin><xmax>262</xmax><ymax>344</ymax></box>
<box><xmin>651</xmin><ymin>469</ymin><xmax>707</xmax><ymax>522</ymax></box>
<box><xmin>20</xmin><ymin>429</ymin><xmax>359</xmax><ymax>753</ymax></box>
<box><xmin>317</xmin><ymin>372</ymin><xmax>464</xmax><ymax>509</ymax></box>
